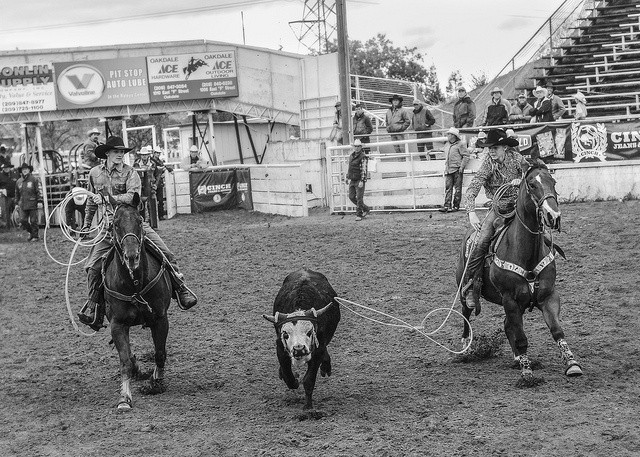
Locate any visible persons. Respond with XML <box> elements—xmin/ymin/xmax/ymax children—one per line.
<box><xmin>0</xmin><ymin>162</ymin><xmax>15</xmax><ymax>229</ymax></box>
<box><xmin>509</xmin><ymin>94</ymin><xmax>534</xmax><ymax>124</ymax></box>
<box><xmin>544</xmin><ymin>83</ymin><xmax>566</xmax><ymax>121</ymax></box>
<box><xmin>346</xmin><ymin>139</ymin><xmax>370</xmax><ymax>221</ymax></box>
<box><xmin>411</xmin><ymin>100</ymin><xmax>435</xmax><ymax>161</ymax></box>
<box><xmin>353</xmin><ymin>105</ymin><xmax>373</xmax><ymax>154</ymax></box>
<box><xmin>77</xmin><ymin>137</ymin><xmax>197</xmax><ymax>331</ymax></box>
<box><xmin>15</xmin><ymin>163</ymin><xmax>43</xmax><ymax>241</ymax></box>
<box><xmin>572</xmin><ymin>92</ymin><xmax>588</xmax><ymax>120</ymax></box>
<box><xmin>453</xmin><ymin>87</ymin><xmax>476</xmax><ymax>127</ymax></box>
<box><xmin>133</xmin><ymin>146</ymin><xmax>160</xmax><ymax>224</ymax></box>
<box><xmin>189</xmin><ymin>144</ymin><xmax>200</xmax><ymax>165</ymax></box>
<box><xmin>82</xmin><ymin>128</ymin><xmax>101</xmax><ymax>168</ymax></box>
<box><xmin>530</xmin><ymin>86</ymin><xmax>556</xmax><ymax>122</ymax></box>
<box><xmin>333</xmin><ymin>102</ymin><xmax>343</xmax><ymax>141</ymax></box>
<box><xmin>386</xmin><ymin>95</ymin><xmax>410</xmax><ymax>159</ymax></box>
<box><xmin>0</xmin><ymin>146</ymin><xmax>11</xmax><ymax>166</ymax></box>
<box><xmin>483</xmin><ymin>87</ymin><xmax>512</xmax><ymax>124</ymax></box>
<box><xmin>438</xmin><ymin>127</ymin><xmax>470</xmax><ymax>212</ymax></box>
<box><xmin>466</xmin><ymin>131</ymin><xmax>487</xmax><ymax>153</ymax></box>
<box><xmin>466</xmin><ymin>129</ymin><xmax>541</xmax><ymax>309</ymax></box>
<box><xmin>151</xmin><ymin>147</ymin><xmax>167</xmax><ymax>221</ymax></box>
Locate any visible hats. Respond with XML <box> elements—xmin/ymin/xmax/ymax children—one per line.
<box><xmin>518</xmin><ymin>93</ymin><xmax>525</xmax><ymax>98</ymax></box>
<box><xmin>458</xmin><ymin>86</ymin><xmax>466</xmax><ymax>92</ymax></box>
<box><xmin>92</xmin><ymin>136</ymin><xmax>135</xmax><ymax>159</ymax></box>
<box><xmin>1</xmin><ymin>146</ymin><xmax>8</xmax><ymax>152</ymax></box>
<box><xmin>543</xmin><ymin>82</ymin><xmax>556</xmax><ymax>91</ymax></box>
<box><xmin>477</xmin><ymin>132</ymin><xmax>487</xmax><ymax>139</ymax></box>
<box><xmin>490</xmin><ymin>86</ymin><xmax>503</xmax><ymax>96</ymax></box>
<box><xmin>388</xmin><ymin>95</ymin><xmax>403</xmax><ymax>102</ymax></box>
<box><xmin>19</xmin><ymin>164</ymin><xmax>33</xmax><ymax>172</ymax></box>
<box><xmin>574</xmin><ymin>92</ymin><xmax>587</xmax><ymax>105</ymax></box>
<box><xmin>136</xmin><ymin>147</ymin><xmax>151</xmax><ymax>155</ymax></box>
<box><xmin>475</xmin><ymin>128</ymin><xmax>519</xmax><ymax>147</ymax></box>
<box><xmin>87</xmin><ymin>128</ymin><xmax>101</xmax><ymax>136</ymax></box>
<box><xmin>188</xmin><ymin>145</ymin><xmax>201</xmax><ymax>152</ymax></box>
<box><xmin>155</xmin><ymin>146</ymin><xmax>162</xmax><ymax>152</ymax></box>
<box><xmin>532</xmin><ymin>86</ymin><xmax>547</xmax><ymax>97</ymax></box>
<box><xmin>335</xmin><ymin>102</ymin><xmax>341</xmax><ymax>107</ymax></box>
<box><xmin>2</xmin><ymin>162</ymin><xmax>15</xmax><ymax>170</ymax></box>
<box><xmin>351</xmin><ymin>139</ymin><xmax>363</xmax><ymax>147</ymax></box>
<box><xmin>444</xmin><ymin>127</ymin><xmax>463</xmax><ymax>140</ymax></box>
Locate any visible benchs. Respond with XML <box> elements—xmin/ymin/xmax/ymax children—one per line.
<box><xmin>593</xmin><ymin>52</ymin><xmax>640</xmax><ymax>63</ymax></box>
<box><xmin>627</xmin><ymin>13</ymin><xmax>640</xmax><ymax>22</ymax></box>
<box><xmin>577</xmin><ymin>13</ymin><xmax>628</xmax><ymax>24</ymax></box>
<box><xmin>601</xmin><ymin>41</ymin><xmax>640</xmax><ymax>52</ymax></box>
<box><xmin>585</xmin><ymin>5</ymin><xmax>635</xmax><ymax>15</ymax></box>
<box><xmin>514</xmin><ymin>85</ymin><xmax>567</xmax><ymax>94</ymax></box>
<box><xmin>533</xmin><ymin>64</ymin><xmax>581</xmax><ymax>73</ymax></box>
<box><xmin>562</xmin><ymin>103</ymin><xmax>640</xmax><ymax>115</ymax></box>
<box><xmin>574</xmin><ymin>71</ymin><xmax>640</xmax><ymax>82</ymax></box>
<box><xmin>560</xmin><ymin>33</ymin><xmax>609</xmax><ymax>44</ymax></box>
<box><xmin>609</xmin><ymin>31</ymin><xmax>640</xmax><ymax>42</ymax></box>
<box><xmin>528</xmin><ymin>74</ymin><xmax>575</xmax><ymax>83</ymax></box>
<box><xmin>565</xmin><ymin>81</ymin><xmax>640</xmax><ymax>93</ymax></box>
<box><xmin>560</xmin><ymin>92</ymin><xmax>640</xmax><ymax>107</ymax></box>
<box><xmin>568</xmin><ymin>23</ymin><xmax>618</xmax><ymax>34</ymax></box>
<box><xmin>584</xmin><ymin>61</ymin><xmax>640</xmax><ymax>74</ymax></box>
<box><xmin>542</xmin><ymin>53</ymin><xmax>590</xmax><ymax>62</ymax></box>
<box><xmin>552</xmin><ymin>43</ymin><xmax>601</xmax><ymax>54</ymax></box>
<box><xmin>619</xmin><ymin>23</ymin><xmax>640</xmax><ymax>33</ymax></box>
<box><xmin>507</xmin><ymin>97</ymin><xmax>537</xmax><ymax>105</ymax></box>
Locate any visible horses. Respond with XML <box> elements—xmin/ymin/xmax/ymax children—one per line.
<box><xmin>98</xmin><ymin>189</ymin><xmax>173</xmax><ymax>411</ymax></box>
<box><xmin>454</xmin><ymin>157</ymin><xmax>584</xmax><ymax>381</ymax></box>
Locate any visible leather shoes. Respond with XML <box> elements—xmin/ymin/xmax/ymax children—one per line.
<box><xmin>463</xmin><ymin>286</ymin><xmax>478</xmax><ymax>311</ymax></box>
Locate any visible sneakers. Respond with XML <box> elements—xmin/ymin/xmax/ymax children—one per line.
<box><xmin>175</xmin><ymin>285</ymin><xmax>197</xmax><ymax>310</ymax></box>
<box><xmin>76</xmin><ymin>307</ymin><xmax>103</xmax><ymax>333</ymax></box>
<box><xmin>362</xmin><ymin>208</ymin><xmax>370</xmax><ymax>216</ymax></box>
<box><xmin>447</xmin><ymin>208</ymin><xmax>458</xmax><ymax>212</ymax></box>
<box><xmin>355</xmin><ymin>217</ymin><xmax>362</xmax><ymax>221</ymax></box>
<box><xmin>438</xmin><ymin>207</ymin><xmax>449</xmax><ymax>212</ymax></box>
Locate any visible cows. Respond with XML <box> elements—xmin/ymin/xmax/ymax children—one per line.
<box><xmin>262</xmin><ymin>266</ymin><xmax>342</xmax><ymax>410</ymax></box>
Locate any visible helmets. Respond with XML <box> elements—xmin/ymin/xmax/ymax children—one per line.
<box><xmin>412</xmin><ymin>99</ymin><xmax>420</xmax><ymax>105</ymax></box>
<box><xmin>505</xmin><ymin>128</ymin><xmax>518</xmax><ymax>139</ymax></box>
